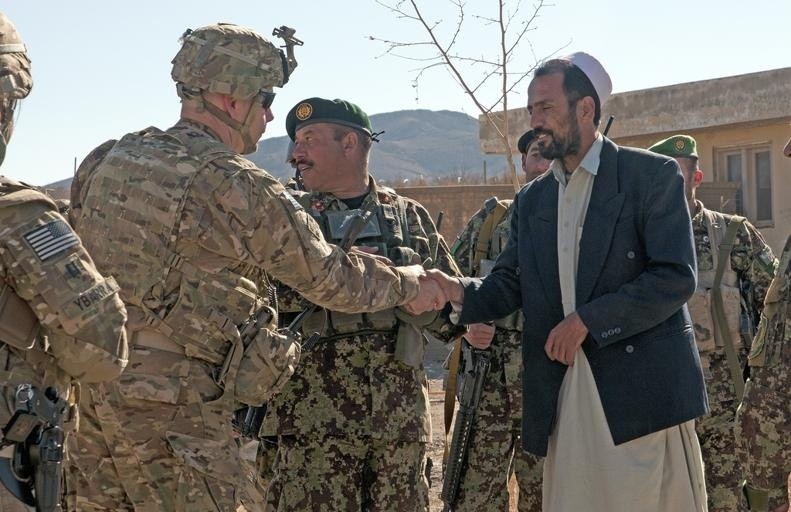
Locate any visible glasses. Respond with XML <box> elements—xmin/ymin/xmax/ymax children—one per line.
<box><xmin>259</xmin><ymin>90</ymin><xmax>275</xmax><ymax>109</ymax></box>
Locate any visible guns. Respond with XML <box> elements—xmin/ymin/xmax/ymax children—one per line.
<box><xmin>16</xmin><ymin>383</ymin><xmax>69</xmax><ymax>512</ymax></box>
<box><xmin>441</xmin><ymin>259</ymin><xmax>496</xmax><ymax>512</ymax></box>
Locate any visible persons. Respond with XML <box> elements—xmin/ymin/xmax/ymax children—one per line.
<box><xmin>733</xmin><ymin>140</ymin><xmax>791</xmax><ymax>512</ymax></box>
<box><xmin>60</xmin><ymin>23</ymin><xmax>446</xmax><ymax>511</ymax></box>
<box><xmin>253</xmin><ymin>97</ymin><xmax>464</xmax><ymax>511</ymax></box>
<box><xmin>1</xmin><ymin>12</ymin><xmax>129</xmax><ymax>512</ymax></box>
<box><xmin>440</xmin><ymin>127</ymin><xmax>554</xmax><ymax>511</ymax></box>
<box><xmin>283</xmin><ymin>141</ymin><xmax>308</xmax><ymax>191</ymax></box>
<box><xmin>426</xmin><ymin>53</ymin><xmax>711</xmax><ymax>512</ymax></box>
<box><xmin>649</xmin><ymin>135</ymin><xmax>780</xmax><ymax>512</ymax></box>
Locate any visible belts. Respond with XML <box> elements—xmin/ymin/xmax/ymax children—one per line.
<box><xmin>124</xmin><ymin>328</ymin><xmax>208</xmax><ymax>366</ymax></box>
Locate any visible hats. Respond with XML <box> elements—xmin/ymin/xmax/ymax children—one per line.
<box><xmin>518</xmin><ymin>128</ymin><xmax>535</xmax><ymax>154</ymax></box>
<box><xmin>648</xmin><ymin>135</ymin><xmax>700</xmax><ymax>159</ymax></box>
<box><xmin>559</xmin><ymin>51</ymin><xmax>614</xmax><ymax>109</ymax></box>
<box><xmin>285</xmin><ymin>97</ymin><xmax>385</xmax><ymax>144</ymax></box>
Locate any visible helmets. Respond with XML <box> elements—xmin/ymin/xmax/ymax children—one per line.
<box><xmin>1</xmin><ymin>11</ymin><xmax>34</xmax><ymax>101</ymax></box>
<box><xmin>170</xmin><ymin>22</ymin><xmax>291</xmax><ymax>100</ymax></box>
<box><xmin>285</xmin><ymin>140</ymin><xmax>297</xmax><ymax>165</ymax></box>
